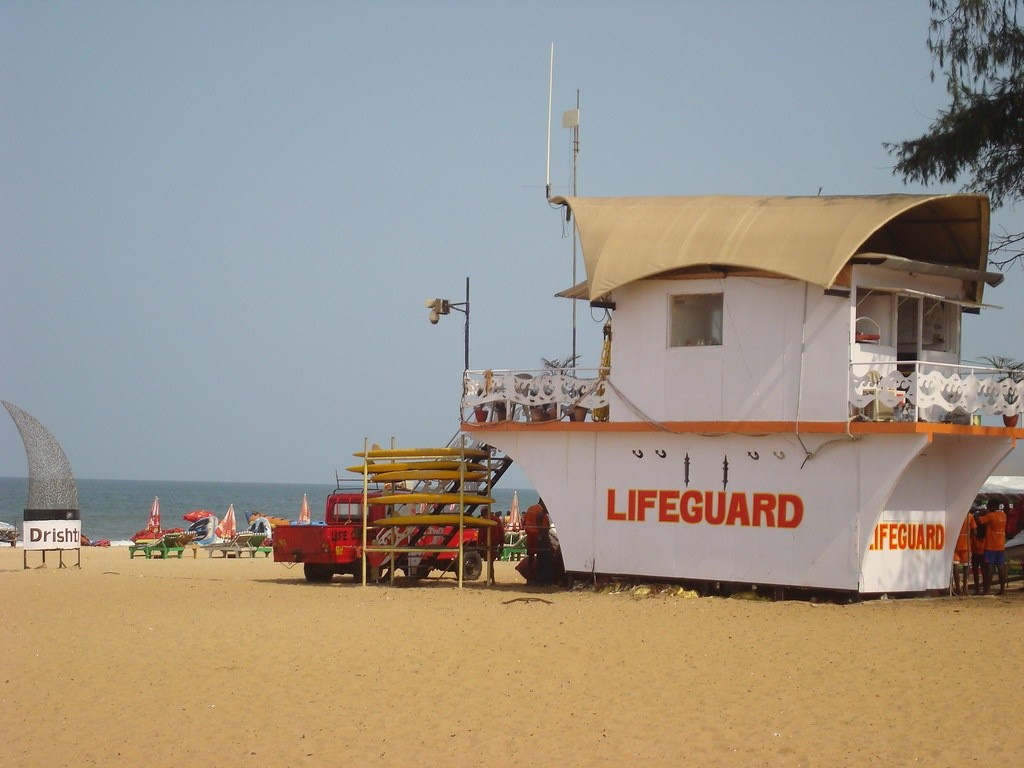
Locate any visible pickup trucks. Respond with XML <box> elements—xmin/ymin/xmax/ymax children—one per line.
<box><xmin>271</xmin><ymin>487</ymin><xmax>484</xmax><ymax>585</ymax></box>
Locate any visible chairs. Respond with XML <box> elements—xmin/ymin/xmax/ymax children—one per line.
<box><xmin>129</xmin><ymin>531</ymin><xmax>272</xmax><ymax>559</ymax></box>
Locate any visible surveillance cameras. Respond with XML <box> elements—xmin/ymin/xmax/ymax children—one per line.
<box><xmin>429</xmin><ymin>309</ymin><xmax>439</xmax><ymax>324</ymax></box>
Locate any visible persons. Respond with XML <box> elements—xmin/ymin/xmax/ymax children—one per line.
<box><xmin>81</xmin><ymin>534</ymin><xmax>90</xmax><ymax>546</ymax></box>
<box><xmin>476</xmin><ymin>511</ymin><xmax>526</xmax><ymax>562</ymax></box>
<box><xmin>477</xmin><ymin>507</ymin><xmax>504</xmax><ymax>584</ymax></box>
<box><xmin>954</xmin><ymin>500</ymin><xmax>1024</xmax><ymax>594</ymax></box>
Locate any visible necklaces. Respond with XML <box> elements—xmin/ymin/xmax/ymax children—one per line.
<box><xmin>524</xmin><ymin>498</ymin><xmax>556</xmax><ymax>587</ymax></box>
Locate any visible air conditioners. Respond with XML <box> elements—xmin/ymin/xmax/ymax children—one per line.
<box><xmin>863</xmin><ymin>388</ymin><xmax>906</xmax><ymax>422</ymax></box>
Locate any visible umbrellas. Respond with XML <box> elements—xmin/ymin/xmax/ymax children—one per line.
<box><xmin>148</xmin><ymin>498</ymin><xmax>162</xmax><ymax>558</ymax></box>
<box><xmin>184</xmin><ymin>510</ymin><xmax>213</xmax><ymax>522</ymax></box>
<box><xmin>214</xmin><ymin>506</ymin><xmax>237</xmax><ymax>558</ymax></box>
<box><xmin>508</xmin><ymin>494</ymin><xmax>522</xmax><ymax>561</ymax></box>
<box><xmin>299</xmin><ymin>495</ymin><xmax>310</xmax><ymax>521</ymax></box>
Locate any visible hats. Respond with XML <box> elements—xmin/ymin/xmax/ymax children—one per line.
<box><xmin>480</xmin><ymin>505</ymin><xmax>489</xmax><ymax>517</ymax></box>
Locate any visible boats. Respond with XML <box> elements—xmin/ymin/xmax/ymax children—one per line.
<box><xmin>457</xmin><ymin>190</ymin><xmax>1024</xmax><ymax>599</ymax></box>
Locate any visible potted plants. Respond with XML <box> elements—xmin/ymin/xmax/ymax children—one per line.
<box><xmin>977</xmin><ymin>354</ymin><xmax>1024</xmax><ymax>427</ymax></box>
<box><xmin>473</xmin><ymin>354</ymin><xmax>589</xmax><ymax>423</ymax></box>
